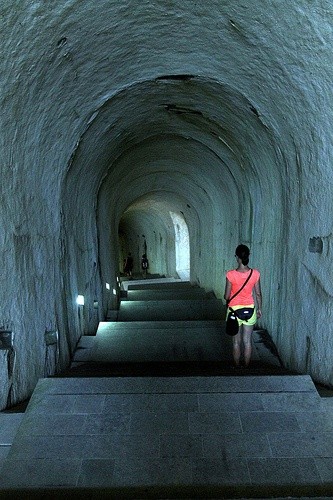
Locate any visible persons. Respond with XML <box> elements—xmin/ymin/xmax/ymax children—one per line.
<box><xmin>225</xmin><ymin>244</ymin><xmax>263</xmax><ymax>371</ymax></box>
<box><xmin>122</xmin><ymin>253</ymin><xmax>134</xmax><ymax>278</ymax></box>
<box><xmin>141</xmin><ymin>255</ymin><xmax>149</xmax><ymax>279</ymax></box>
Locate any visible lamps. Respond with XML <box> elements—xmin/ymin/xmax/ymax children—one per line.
<box><xmin>43</xmin><ymin>330</ymin><xmax>59</xmax><ymax>346</ymax></box>
<box><xmin>0</xmin><ymin>330</ymin><xmax>15</xmax><ymax>350</ymax></box>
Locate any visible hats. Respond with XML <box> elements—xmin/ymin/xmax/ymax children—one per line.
<box><xmin>225</xmin><ymin>311</ymin><xmax>239</xmax><ymax>336</ymax></box>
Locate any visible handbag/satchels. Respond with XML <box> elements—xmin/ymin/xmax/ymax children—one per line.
<box><xmin>229</xmin><ymin>307</ymin><xmax>255</xmax><ymax>320</ymax></box>
